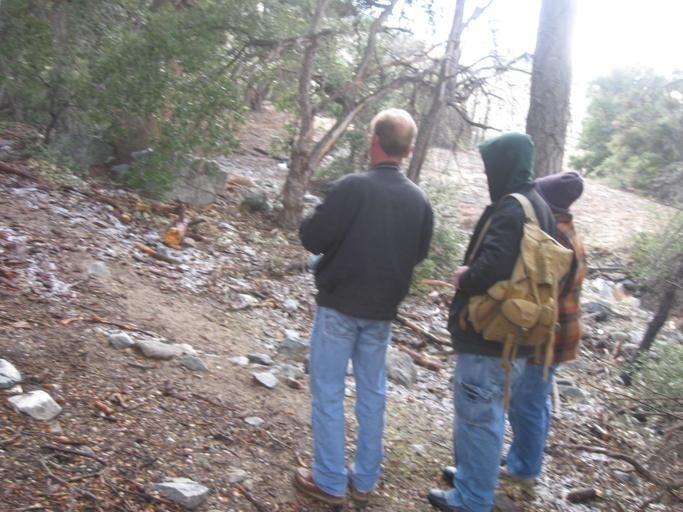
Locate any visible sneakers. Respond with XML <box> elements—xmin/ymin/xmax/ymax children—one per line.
<box><xmin>442</xmin><ymin>465</ymin><xmax>457</xmax><ymax>487</ymax></box>
<box><xmin>347</xmin><ymin>462</ymin><xmax>370</xmax><ymax>502</ymax></box>
<box><xmin>427</xmin><ymin>488</ymin><xmax>453</xmax><ymax>512</ymax></box>
<box><xmin>498</xmin><ymin>465</ymin><xmax>536</xmax><ymax>487</ymax></box>
<box><xmin>292</xmin><ymin>466</ymin><xmax>345</xmax><ymax>504</ymax></box>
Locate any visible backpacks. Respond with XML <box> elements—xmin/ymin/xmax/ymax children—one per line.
<box><xmin>458</xmin><ymin>192</ymin><xmax>575</xmax><ymax>410</ymax></box>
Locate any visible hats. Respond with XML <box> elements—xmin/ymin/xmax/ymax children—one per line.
<box><xmin>533</xmin><ymin>171</ymin><xmax>583</xmax><ymax>214</ymax></box>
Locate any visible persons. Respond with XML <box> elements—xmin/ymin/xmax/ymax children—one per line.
<box><xmin>426</xmin><ymin>130</ymin><xmax>558</xmax><ymax>512</ymax></box>
<box><xmin>293</xmin><ymin>108</ymin><xmax>433</xmax><ymax>505</ymax></box>
<box><xmin>498</xmin><ymin>171</ymin><xmax>587</xmax><ymax>487</ymax></box>
